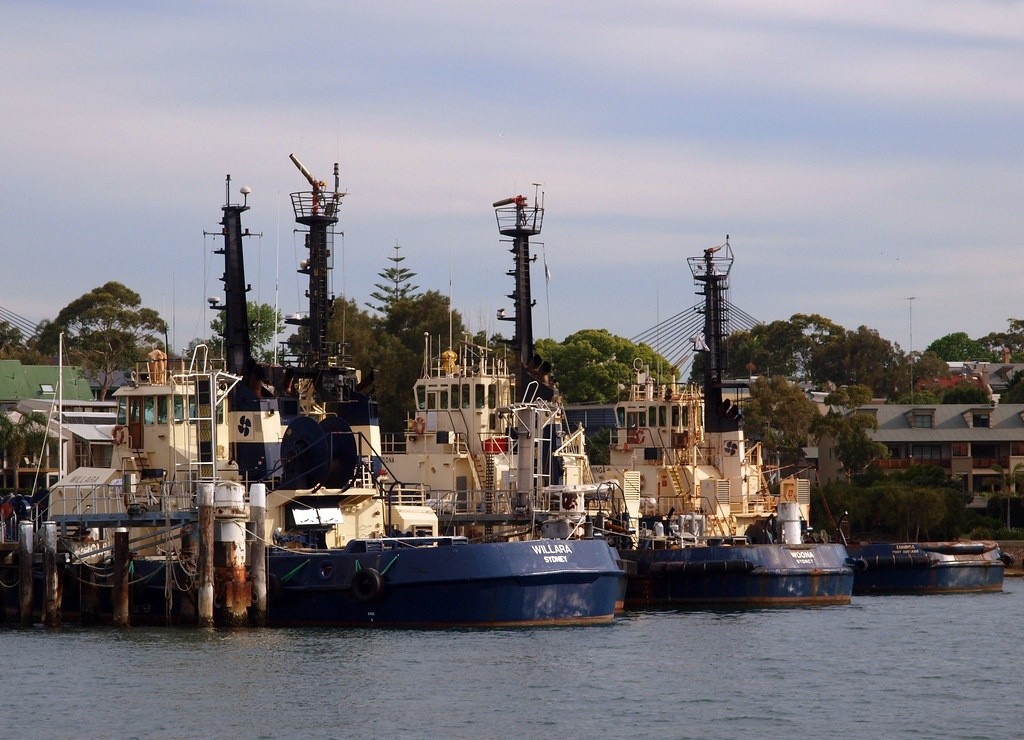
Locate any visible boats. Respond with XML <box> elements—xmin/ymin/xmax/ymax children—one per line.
<box><xmin>0</xmin><ymin>192</ymin><xmax>1017</xmax><ymax>632</ymax></box>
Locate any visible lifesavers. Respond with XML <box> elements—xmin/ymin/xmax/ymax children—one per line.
<box><xmin>112</xmin><ymin>426</ymin><xmax>124</xmax><ymax>445</ymax></box>
<box><xmin>634</xmin><ymin>429</ymin><xmax>646</xmax><ymax>444</ymax></box>
